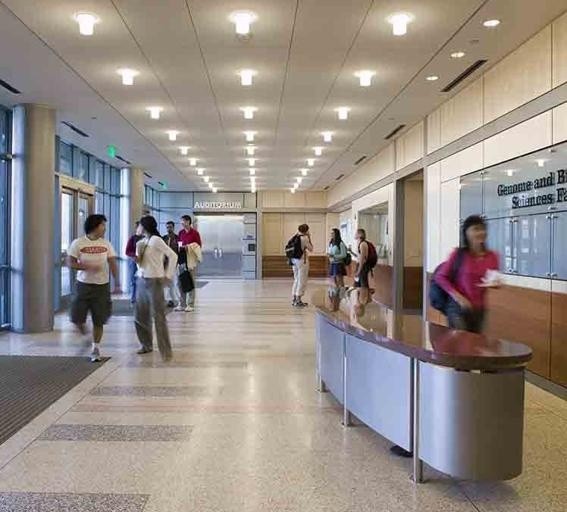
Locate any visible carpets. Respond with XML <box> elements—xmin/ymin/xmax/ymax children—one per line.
<box><xmin>191</xmin><ymin>280</ymin><xmax>209</xmax><ymax>290</ymax></box>
<box><xmin>60</xmin><ymin>299</ymin><xmax>179</xmax><ymax>317</ymax></box>
<box><xmin>0</xmin><ymin>355</ymin><xmax>123</xmax><ymax>471</ymax></box>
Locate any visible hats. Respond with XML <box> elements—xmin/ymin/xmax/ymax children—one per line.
<box><xmin>140</xmin><ymin>216</ymin><xmax>157</xmax><ymax>233</ymax></box>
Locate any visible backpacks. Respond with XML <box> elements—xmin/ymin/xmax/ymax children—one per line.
<box><xmin>429</xmin><ymin>247</ymin><xmax>463</xmax><ymax>316</ymax></box>
<box><xmin>285</xmin><ymin>233</ymin><xmax>307</xmax><ymax>266</ymax></box>
<box><xmin>358</xmin><ymin>239</ymin><xmax>377</xmax><ymax>278</ymax></box>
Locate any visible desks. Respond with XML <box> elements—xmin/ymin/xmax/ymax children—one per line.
<box><xmin>311</xmin><ymin>291</ymin><xmax>534</xmax><ymax>484</ymax></box>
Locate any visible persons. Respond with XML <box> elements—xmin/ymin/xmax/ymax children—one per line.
<box><xmin>445</xmin><ymin>215</ymin><xmax>502</xmax><ymax>373</ymax></box>
<box><xmin>66</xmin><ymin>214</ymin><xmax>123</xmax><ymax>362</ymax></box>
<box><xmin>173</xmin><ymin>215</ymin><xmax>202</xmax><ymax>312</ymax></box>
<box><xmin>290</xmin><ymin>224</ymin><xmax>313</xmax><ymax>308</ymax></box>
<box><xmin>326</xmin><ymin>228</ymin><xmax>347</xmax><ymax>283</ymax></box>
<box><xmin>347</xmin><ymin>229</ymin><xmax>369</xmax><ymax>288</ymax></box>
<box><xmin>327</xmin><ymin>283</ymin><xmax>346</xmax><ymax>312</ymax></box>
<box><xmin>160</xmin><ymin>217</ymin><xmax>178</xmax><ymax>252</ymax></box>
<box><xmin>353</xmin><ymin>288</ymin><xmax>369</xmax><ymax>317</ymax></box>
<box><xmin>125</xmin><ymin>221</ymin><xmax>145</xmax><ymax>304</ymax></box>
<box><xmin>133</xmin><ymin>216</ymin><xmax>178</xmax><ymax>363</ymax></box>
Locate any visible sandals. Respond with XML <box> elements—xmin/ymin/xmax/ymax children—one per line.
<box><xmin>294</xmin><ymin>300</ymin><xmax>308</xmax><ymax>309</ymax></box>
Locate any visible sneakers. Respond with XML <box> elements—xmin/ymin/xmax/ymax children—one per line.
<box><xmin>173</xmin><ymin>305</ymin><xmax>184</xmax><ymax>310</ymax></box>
<box><xmin>91</xmin><ymin>347</ymin><xmax>100</xmax><ymax>362</ymax></box>
<box><xmin>184</xmin><ymin>307</ymin><xmax>193</xmax><ymax>311</ymax></box>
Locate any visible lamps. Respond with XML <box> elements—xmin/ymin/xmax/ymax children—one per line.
<box><xmin>386</xmin><ymin>12</ymin><xmax>415</xmax><ymax>36</ymax></box>
<box><xmin>245</xmin><ymin>145</ymin><xmax>256</xmax><ymax>154</ymax></box>
<box><xmin>290</xmin><ymin>177</ymin><xmax>303</xmax><ymax>195</ymax></box>
<box><xmin>235</xmin><ymin>69</ymin><xmax>256</xmax><ymax>87</ymax></box>
<box><xmin>319</xmin><ymin>129</ymin><xmax>333</xmax><ymax>141</ymax></box>
<box><xmin>312</xmin><ymin>146</ymin><xmax>324</xmax><ymax>156</ymax></box>
<box><xmin>196</xmin><ymin>168</ymin><xmax>205</xmax><ymax>175</ymax></box>
<box><xmin>165</xmin><ymin>129</ymin><xmax>180</xmax><ymax>140</ymax></box>
<box><xmin>204</xmin><ymin>175</ymin><xmax>218</xmax><ymax>194</ymax></box>
<box><xmin>73</xmin><ymin>11</ymin><xmax>99</xmax><ymax>37</ymax></box>
<box><xmin>244</xmin><ymin>158</ymin><xmax>257</xmax><ymax>192</ymax></box>
<box><xmin>305</xmin><ymin>158</ymin><xmax>316</xmax><ymax>165</ymax></box>
<box><xmin>188</xmin><ymin>157</ymin><xmax>199</xmax><ymax>166</ymax></box>
<box><xmin>239</xmin><ymin>107</ymin><xmax>257</xmax><ymax>118</ymax></box>
<box><xmin>179</xmin><ymin>146</ymin><xmax>188</xmax><ymax>156</ymax></box>
<box><xmin>333</xmin><ymin>106</ymin><xmax>349</xmax><ymax>119</ymax></box>
<box><xmin>116</xmin><ymin>67</ymin><xmax>139</xmax><ymax>87</ymax></box>
<box><xmin>230</xmin><ymin>9</ymin><xmax>256</xmax><ymax>36</ymax></box>
<box><xmin>354</xmin><ymin>69</ymin><xmax>376</xmax><ymax>86</ymax></box>
<box><xmin>242</xmin><ymin>130</ymin><xmax>258</xmax><ymax>142</ymax></box>
<box><xmin>300</xmin><ymin>168</ymin><xmax>309</xmax><ymax>176</ymax></box>
<box><xmin>145</xmin><ymin>106</ymin><xmax>163</xmax><ymax>120</ymax></box>
